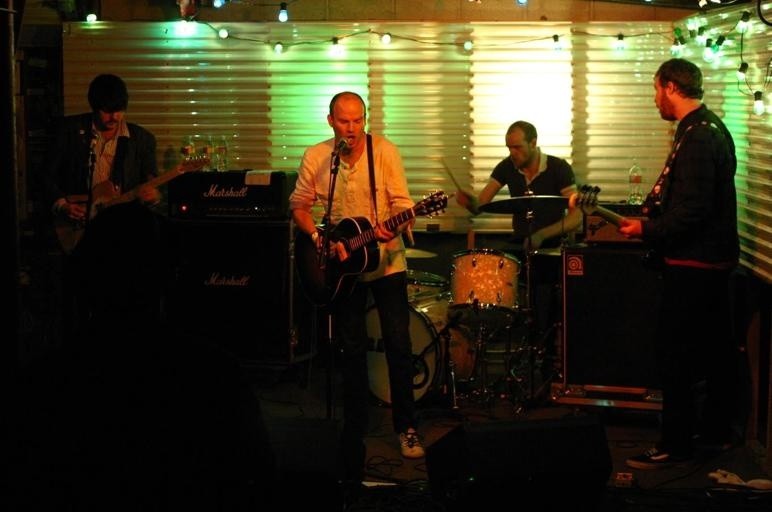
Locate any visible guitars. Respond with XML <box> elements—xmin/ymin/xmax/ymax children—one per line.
<box><xmin>305</xmin><ymin>187</ymin><xmax>451</xmax><ymax>308</ymax></box>
<box><xmin>59</xmin><ymin>161</ymin><xmax>194</xmax><ymax>257</ymax></box>
<box><xmin>577</xmin><ymin>188</ymin><xmax>630</xmax><ymax>234</ymax></box>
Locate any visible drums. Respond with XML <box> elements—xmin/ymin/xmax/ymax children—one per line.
<box><xmin>449</xmin><ymin>246</ymin><xmax>520</xmax><ymax>327</ymax></box>
<box><xmin>406</xmin><ymin>269</ymin><xmax>445</xmax><ymax>304</ymax></box>
<box><xmin>357</xmin><ymin>294</ymin><xmax>448</xmax><ymax>408</ymax></box>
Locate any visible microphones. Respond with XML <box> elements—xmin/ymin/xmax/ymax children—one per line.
<box><xmin>331</xmin><ymin>137</ymin><xmax>349</xmax><ymax>156</ymax></box>
<box><xmin>89</xmin><ymin>134</ymin><xmax>100</xmax><ymax>154</ymax></box>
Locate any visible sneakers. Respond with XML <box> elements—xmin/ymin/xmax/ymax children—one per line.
<box><xmin>625</xmin><ymin>446</ymin><xmax>696</xmax><ymax>470</ymax></box>
<box><xmin>399</xmin><ymin>427</ymin><xmax>425</xmax><ymax>459</ymax></box>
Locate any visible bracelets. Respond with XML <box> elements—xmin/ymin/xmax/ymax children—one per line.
<box><xmin>310</xmin><ymin>230</ymin><xmax>319</xmax><ymax>242</ymax></box>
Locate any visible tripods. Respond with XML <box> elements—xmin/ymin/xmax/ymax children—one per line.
<box><xmin>419</xmin><ymin>218</ymin><xmax>558</xmax><ymax>420</ymax></box>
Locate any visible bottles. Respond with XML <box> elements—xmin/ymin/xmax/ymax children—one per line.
<box><xmin>180</xmin><ymin>135</ymin><xmax>195</xmax><ymax>159</ymax></box>
<box><xmin>202</xmin><ymin>133</ymin><xmax>229</xmax><ymax>174</ymax></box>
<box><xmin>627</xmin><ymin>157</ymin><xmax>645</xmax><ymax>208</ymax></box>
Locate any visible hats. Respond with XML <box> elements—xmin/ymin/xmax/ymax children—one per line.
<box><xmin>88</xmin><ymin>74</ymin><xmax>129</xmax><ymax>112</ymax></box>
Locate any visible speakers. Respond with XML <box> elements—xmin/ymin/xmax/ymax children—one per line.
<box><xmin>563</xmin><ymin>245</ymin><xmax>666</xmax><ymax>390</ymax></box>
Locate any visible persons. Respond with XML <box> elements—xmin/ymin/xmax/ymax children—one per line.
<box><xmin>37</xmin><ymin>72</ymin><xmax>161</xmax><ymax>221</ymax></box>
<box><xmin>456</xmin><ymin>121</ymin><xmax>583</xmax><ymax>249</ymax></box>
<box><xmin>617</xmin><ymin>59</ymin><xmax>740</xmax><ymax>470</ymax></box>
<box><xmin>286</xmin><ymin>91</ymin><xmax>425</xmax><ymax>458</ymax></box>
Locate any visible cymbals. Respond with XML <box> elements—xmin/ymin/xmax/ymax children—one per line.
<box><xmin>478</xmin><ymin>191</ymin><xmax>568</xmax><ymax>215</ymax></box>
<box><xmin>404</xmin><ymin>246</ymin><xmax>440</xmax><ymax>262</ymax></box>
